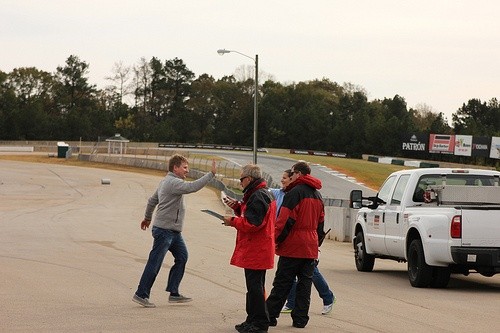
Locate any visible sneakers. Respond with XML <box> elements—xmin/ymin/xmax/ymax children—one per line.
<box><xmin>280</xmin><ymin>304</ymin><xmax>292</xmax><ymax>313</ymax></box>
<box><xmin>321</xmin><ymin>292</ymin><xmax>336</xmax><ymax>315</ymax></box>
<box><xmin>132</xmin><ymin>294</ymin><xmax>156</xmax><ymax>307</ymax></box>
<box><xmin>168</xmin><ymin>294</ymin><xmax>192</xmax><ymax>303</ymax></box>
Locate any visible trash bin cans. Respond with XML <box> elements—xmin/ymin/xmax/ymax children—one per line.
<box><xmin>58</xmin><ymin>146</ymin><xmax>68</xmax><ymax>158</ymax></box>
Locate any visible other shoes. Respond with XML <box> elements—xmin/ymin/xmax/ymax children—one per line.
<box><xmin>235</xmin><ymin>321</ymin><xmax>249</xmax><ymax>331</ymax></box>
<box><xmin>238</xmin><ymin>325</ymin><xmax>267</xmax><ymax>333</ymax></box>
<box><xmin>269</xmin><ymin>320</ymin><xmax>277</xmax><ymax>326</ymax></box>
<box><xmin>294</xmin><ymin>323</ymin><xmax>305</xmax><ymax>328</ymax></box>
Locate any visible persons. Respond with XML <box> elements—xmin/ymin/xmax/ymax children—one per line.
<box><xmin>264</xmin><ymin>161</ymin><xmax>325</xmax><ymax>328</ymax></box>
<box><xmin>222</xmin><ymin>164</ymin><xmax>277</xmax><ymax>333</ymax></box>
<box><xmin>132</xmin><ymin>154</ymin><xmax>216</xmax><ymax>307</ymax></box>
<box><xmin>269</xmin><ymin>167</ymin><xmax>337</xmax><ymax>315</ymax></box>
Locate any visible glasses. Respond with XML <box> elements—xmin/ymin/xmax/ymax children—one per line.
<box><xmin>240</xmin><ymin>175</ymin><xmax>252</xmax><ymax>183</ymax></box>
<box><xmin>288</xmin><ymin>172</ymin><xmax>297</xmax><ymax>177</ymax></box>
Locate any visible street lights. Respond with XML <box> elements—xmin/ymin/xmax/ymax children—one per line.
<box><xmin>217</xmin><ymin>48</ymin><xmax>258</xmax><ymax>166</ymax></box>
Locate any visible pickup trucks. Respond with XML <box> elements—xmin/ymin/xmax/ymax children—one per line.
<box><xmin>349</xmin><ymin>165</ymin><xmax>496</xmax><ymax>289</ymax></box>
